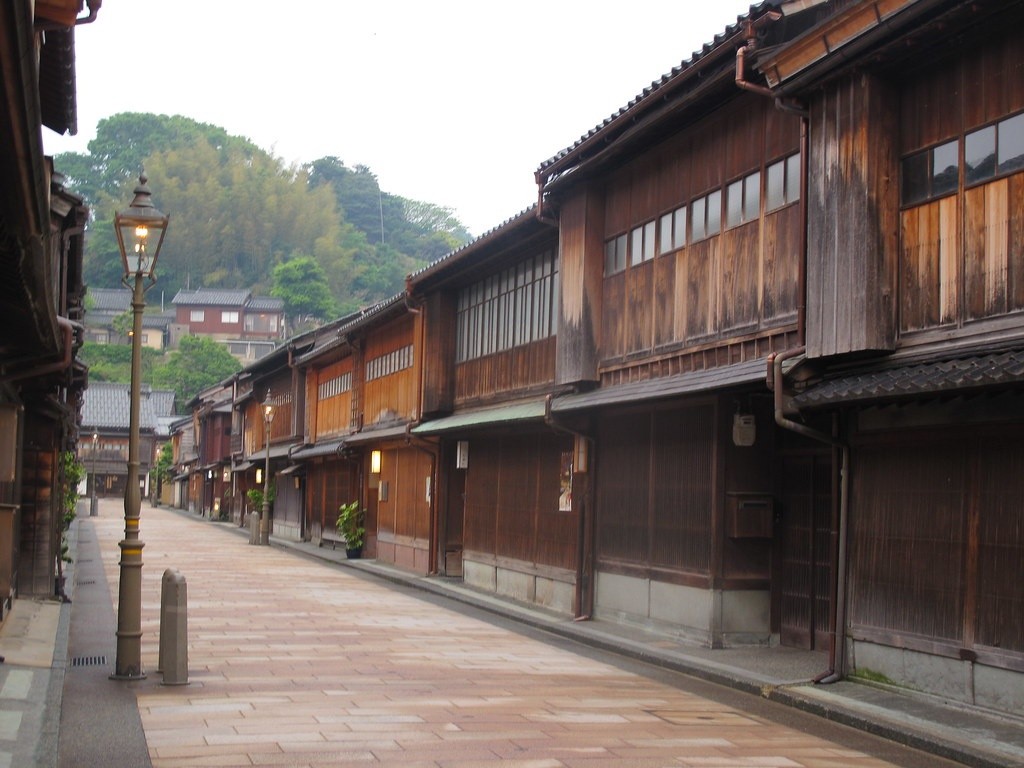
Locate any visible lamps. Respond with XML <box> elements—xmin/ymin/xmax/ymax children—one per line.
<box><xmin>456</xmin><ymin>440</ymin><xmax>468</xmax><ymax>469</ymax></box>
<box><xmin>371</xmin><ymin>447</ymin><xmax>381</xmax><ymax>475</ymax></box>
<box><xmin>574</xmin><ymin>431</ymin><xmax>587</xmax><ymax>473</ymax></box>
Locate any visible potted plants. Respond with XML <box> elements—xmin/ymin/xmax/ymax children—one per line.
<box><xmin>336</xmin><ymin>501</ymin><xmax>370</xmax><ymax>559</ymax></box>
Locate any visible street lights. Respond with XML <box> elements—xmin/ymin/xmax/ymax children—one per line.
<box><xmin>256</xmin><ymin>388</ymin><xmax>280</xmax><ymax>546</ymax></box>
<box><xmin>107</xmin><ymin>167</ymin><xmax>173</xmax><ymax>680</ymax></box>
<box><xmin>155</xmin><ymin>443</ymin><xmax>163</xmax><ymax>503</ymax></box>
<box><xmin>90</xmin><ymin>427</ymin><xmax>101</xmax><ymax>516</ymax></box>
